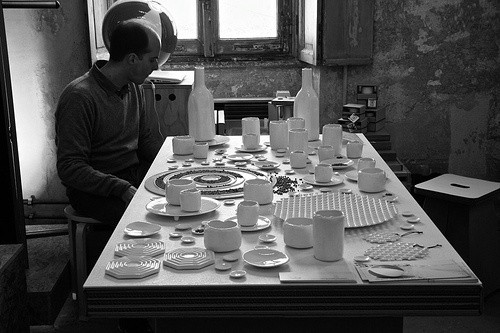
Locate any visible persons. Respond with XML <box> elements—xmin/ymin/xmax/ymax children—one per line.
<box><xmin>55</xmin><ymin>19</ymin><xmax>162</xmax><ymax>229</ymax></box>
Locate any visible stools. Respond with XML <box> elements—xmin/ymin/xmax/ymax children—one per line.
<box><xmin>63</xmin><ymin>205</ymin><xmax>104</xmax><ymax>310</ymax></box>
<box><xmin>413</xmin><ymin>172</ymin><xmax>500</xmax><ymax>300</ymax></box>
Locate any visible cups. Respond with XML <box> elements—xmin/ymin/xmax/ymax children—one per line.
<box><xmin>318</xmin><ymin>146</ymin><xmax>333</xmax><ymax>161</ymax></box>
<box><xmin>357</xmin><ymin>168</ymin><xmax>386</xmax><ymax>193</ymax></box>
<box><xmin>312</xmin><ymin>210</ymin><xmax>345</xmax><ymax>261</ymax></box>
<box><xmin>346</xmin><ymin>141</ymin><xmax>363</xmax><ymax>159</ymax></box>
<box><xmin>237</xmin><ymin>199</ymin><xmax>258</xmax><ymax>227</ymax></box>
<box><xmin>284</xmin><ymin>218</ymin><xmax>314</xmax><ymax>249</ymax></box>
<box><xmin>315</xmin><ymin>163</ymin><xmax>332</xmax><ymax>182</ymax></box>
<box><xmin>179</xmin><ymin>189</ymin><xmax>201</xmax><ymax>211</ymax></box>
<box><xmin>357</xmin><ymin>157</ymin><xmax>376</xmax><ymax>170</ymax></box>
<box><xmin>172</xmin><ymin>136</ymin><xmax>208</xmax><ymax>158</ymax></box>
<box><xmin>289</xmin><ymin>150</ymin><xmax>306</xmax><ymax>168</ymax></box>
<box><xmin>165</xmin><ymin>179</ymin><xmax>196</xmax><ymax>206</ymax></box>
<box><xmin>322</xmin><ymin>124</ymin><xmax>342</xmax><ymax>153</ymax></box>
<box><xmin>244</xmin><ymin>180</ymin><xmax>273</xmax><ymax>205</ymax></box>
<box><xmin>241</xmin><ymin>117</ymin><xmax>307</xmax><ymax>152</ymax></box>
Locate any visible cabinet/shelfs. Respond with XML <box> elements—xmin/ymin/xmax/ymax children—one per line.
<box><xmin>143</xmin><ymin>70</ymin><xmax>196</xmax><ymax>137</ymax></box>
<box><xmin>296</xmin><ymin>0</ymin><xmax>375</xmax><ymax>67</ymax></box>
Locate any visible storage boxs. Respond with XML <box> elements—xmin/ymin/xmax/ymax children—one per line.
<box><xmin>338</xmin><ymin>84</ymin><xmax>403</xmax><ymax>171</ymax></box>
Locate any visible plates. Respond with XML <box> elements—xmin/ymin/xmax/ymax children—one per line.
<box><xmin>228</xmin><ymin>145</ymin><xmax>282</xmax><ymax>169</ymax></box>
<box><xmin>145</xmin><ymin>198</ymin><xmax>220</xmax><ymax>221</ymax></box>
<box><xmin>242</xmin><ymin>249</ymin><xmax>290</xmax><ymax>267</ymax></box>
<box><xmin>302</xmin><ymin>175</ymin><xmax>343</xmax><ymax>186</ymax></box>
<box><xmin>124</xmin><ymin>221</ymin><xmax>161</xmax><ymax>236</ymax></box>
<box><xmin>225</xmin><ymin>216</ymin><xmax>271</xmax><ymax>231</ymax></box>
<box><xmin>321</xmin><ymin>159</ymin><xmax>353</xmax><ymax>169</ymax></box>
<box><xmin>196</xmin><ymin>135</ymin><xmax>229</xmax><ymax>146</ymax></box>
<box><xmin>345</xmin><ymin>170</ymin><xmax>361</xmax><ymax>181</ymax></box>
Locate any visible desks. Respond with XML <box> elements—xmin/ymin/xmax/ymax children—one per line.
<box><xmin>81</xmin><ymin>133</ymin><xmax>484</xmax><ymax>333</ymax></box>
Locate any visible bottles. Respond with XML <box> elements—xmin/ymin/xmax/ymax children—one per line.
<box><xmin>204</xmin><ymin>220</ymin><xmax>241</xmax><ymax>252</ymax></box>
<box><xmin>294</xmin><ymin>69</ymin><xmax>319</xmax><ymax>141</ymax></box>
<box><xmin>187</xmin><ymin>66</ymin><xmax>215</xmax><ymax>142</ymax></box>
